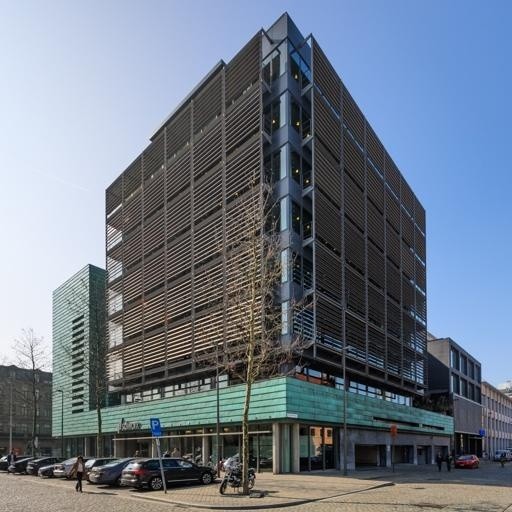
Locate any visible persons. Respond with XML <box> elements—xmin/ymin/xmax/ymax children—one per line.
<box><xmin>445</xmin><ymin>450</ymin><xmax>454</xmax><ymax>472</ymax></box>
<box><xmin>171</xmin><ymin>447</ymin><xmax>181</xmax><ymax>458</ymax></box>
<box><xmin>72</xmin><ymin>456</ymin><xmax>86</xmax><ymax>494</ymax></box>
<box><xmin>500</xmin><ymin>451</ymin><xmax>506</xmax><ymax>468</ymax></box>
<box><xmin>434</xmin><ymin>450</ymin><xmax>444</xmax><ymax>473</ymax></box>
<box><xmin>163</xmin><ymin>450</ymin><xmax>172</xmax><ymax>457</ymax></box>
<box><xmin>133</xmin><ymin>450</ymin><xmax>143</xmax><ymax>458</ymax></box>
<box><xmin>7</xmin><ymin>449</ymin><xmax>17</xmax><ymax>475</ymax></box>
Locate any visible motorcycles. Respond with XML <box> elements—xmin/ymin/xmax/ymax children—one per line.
<box><xmin>219</xmin><ymin>453</ymin><xmax>257</xmax><ymax>495</ymax></box>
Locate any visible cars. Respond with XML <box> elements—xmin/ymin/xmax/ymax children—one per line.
<box><xmin>0</xmin><ymin>454</ymin><xmax>218</xmax><ymax>491</ymax></box>
<box><xmin>455</xmin><ymin>454</ymin><xmax>480</xmax><ymax>469</ymax></box>
